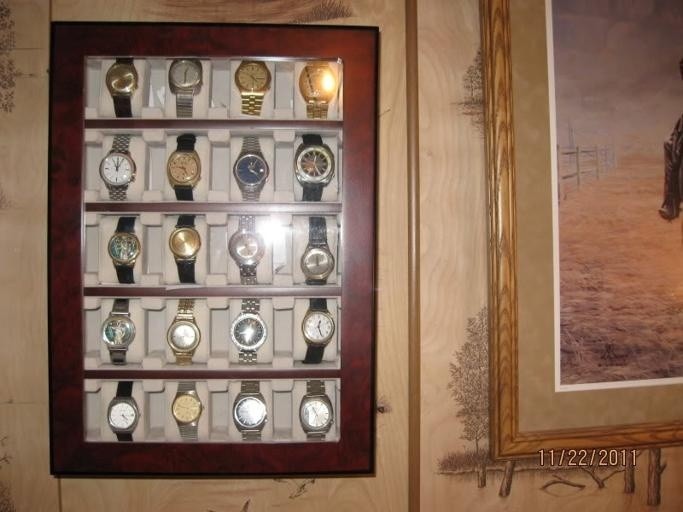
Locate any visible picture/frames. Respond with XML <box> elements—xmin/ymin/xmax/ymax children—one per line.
<box><xmin>475</xmin><ymin>0</ymin><xmax>683</xmax><ymax>462</ymax></box>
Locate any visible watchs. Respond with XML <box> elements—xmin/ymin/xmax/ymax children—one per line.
<box><xmin>229</xmin><ymin>297</ymin><xmax>268</xmax><ymax>365</ymax></box>
<box><xmin>298</xmin><ymin>380</ymin><xmax>335</xmax><ymax>442</ymax></box>
<box><xmin>167</xmin><ymin>59</ymin><xmax>203</xmax><ymax>119</ymax></box>
<box><xmin>231</xmin><ymin>136</ymin><xmax>270</xmax><ymax>201</ymax></box>
<box><xmin>105</xmin><ymin>57</ymin><xmax>138</xmax><ymax>119</ymax></box>
<box><xmin>107</xmin><ymin>381</ymin><xmax>141</xmax><ymax>441</ymax></box>
<box><xmin>107</xmin><ymin>216</ymin><xmax>141</xmax><ymax>283</ymax></box>
<box><xmin>228</xmin><ymin>215</ymin><xmax>266</xmax><ymax>285</ymax></box>
<box><xmin>168</xmin><ymin>215</ymin><xmax>201</xmax><ymax>284</ymax></box>
<box><xmin>234</xmin><ymin>60</ymin><xmax>271</xmax><ymax>116</ymax></box>
<box><xmin>231</xmin><ymin>380</ymin><xmax>268</xmax><ymax>442</ymax></box>
<box><xmin>98</xmin><ymin>299</ymin><xmax>136</xmax><ymax>366</ymax></box>
<box><xmin>299</xmin><ymin>297</ymin><xmax>335</xmax><ymax>364</ymax></box>
<box><xmin>169</xmin><ymin>381</ymin><xmax>206</xmax><ymax>440</ymax></box>
<box><xmin>298</xmin><ymin>62</ymin><xmax>339</xmax><ymax>119</ymax></box>
<box><xmin>299</xmin><ymin>216</ymin><xmax>334</xmax><ymax>284</ymax></box>
<box><xmin>166</xmin><ymin>298</ymin><xmax>200</xmax><ymax>365</ymax></box>
<box><xmin>98</xmin><ymin>134</ymin><xmax>136</xmax><ymax>201</ymax></box>
<box><xmin>165</xmin><ymin>133</ymin><xmax>202</xmax><ymax>201</ymax></box>
<box><xmin>293</xmin><ymin>134</ymin><xmax>336</xmax><ymax>202</ymax></box>
